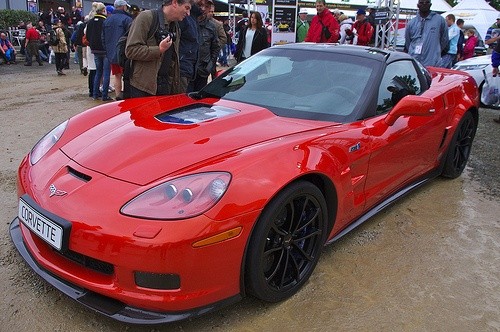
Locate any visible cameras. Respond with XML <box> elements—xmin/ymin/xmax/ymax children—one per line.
<box><xmin>154</xmin><ymin>30</ymin><xmax>177</xmax><ymax>44</ymax></box>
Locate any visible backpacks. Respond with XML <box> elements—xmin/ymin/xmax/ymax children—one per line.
<box><xmin>117</xmin><ymin>9</ymin><xmax>157</xmax><ymax>68</ymax></box>
<box><xmin>47</xmin><ymin>27</ymin><xmax>60</xmax><ymax>46</ymax></box>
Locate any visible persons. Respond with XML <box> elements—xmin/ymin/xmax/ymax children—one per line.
<box><xmin>452</xmin><ymin>19</ymin><xmax>464</xmax><ymax>67</ymax></box>
<box><xmin>442</xmin><ymin>14</ymin><xmax>460</xmax><ymax>68</ymax></box>
<box><xmin>405</xmin><ymin>0</ymin><xmax>449</xmax><ymax>66</ymax></box>
<box><xmin>0</xmin><ymin>0</ymin><xmax>272</xmax><ymax>100</ymax></box>
<box><xmin>462</xmin><ymin>30</ymin><xmax>478</xmax><ymax>60</ymax></box>
<box><xmin>304</xmin><ymin>0</ymin><xmax>340</xmax><ymax>43</ymax></box>
<box><xmin>485</xmin><ymin>14</ymin><xmax>500</xmax><ymax>77</ymax></box>
<box><xmin>353</xmin><ymin>9</ymin><xmax>374</xmax><ymax>46</ymax></box>
<box><xmin>366</xmin><ymin>8</ymin><xmax>375</xmax><ymax>25</ymax></box>
<box><xmin>296</xmin><ymin>11</ymin><xmax>309</xmax><ymax>42</ymax></box>
<box><xmin>337</xmin><ymin>14</ymin><xmax>358</xmax><ymax>45</ymax></box>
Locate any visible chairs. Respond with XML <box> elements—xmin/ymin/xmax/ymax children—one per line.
<box><xmin>284</xmin><ymin>58</ymin><xmax>335</xmax><ymax>97</ymax></box>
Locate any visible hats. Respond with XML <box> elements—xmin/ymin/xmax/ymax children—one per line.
<box><xmin>298</xmin><ymin>8</ymin><xmax>308</xmax><ymax>14</ymax></box>
<box><xmin>356</xmin><ymin>9</ymin><xmax>366</xmax><ymax>15</ymax></box>
<box><xmin>366</xmin><ymin>3</ymin><xmax>378</xmax><ymax>10</ymax></box>
<box><xmin>114</xmin><ymin>0</ymin><xmax>127</xmax><ymax>7</ymax></box>
<box><xmin>106</xmin><ymin>5</ymin><xmax>115</xmax><ymax>14</ymax></box>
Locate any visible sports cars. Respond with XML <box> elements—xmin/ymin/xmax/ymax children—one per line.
<box><xmin>9</xmin><ymin>41</ymin><xmax>481</xmax><ymax>325</ymax></box>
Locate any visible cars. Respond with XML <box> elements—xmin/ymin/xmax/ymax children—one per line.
<box><xmin>450</xmin><ymin>53</ymin><xmax>500</xmax><ymax>110</ymax></box>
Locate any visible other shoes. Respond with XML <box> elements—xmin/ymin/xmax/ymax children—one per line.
<box><xmin>102</xmin><ymin>96</ymin><xmax>114</xmax><ymax>101</ymax></box>
<box><xmin>493</xmin><ymin>114</ymin><xmax>500</xmax><ymax>122</ymax></box>
<box><xmin>39</xmin><ymin>62</ymin><xmax>43</xmax><ymax>66</ymax></box>
<box><xmin>24</xmin><ymin>62</ymin><xmax>32</xmax><ymax>66</ymax></box>
<box><xmin>99</xmin><ymin>82</ymin><xmax>115</xmax><ymax>92</ymax></box>
<box><xmin>57</xmin><ymin>68</ymin><xmax>65</xmax><ymax>76</ymax></box>
<box><xmin>93</xmin><ymin>95</ymin><xmax>102</xmax><ymax>101</ymax></box>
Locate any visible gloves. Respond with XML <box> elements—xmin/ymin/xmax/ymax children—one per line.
<box><xmin>344</xmin><ymin>28</ymin><xmax>355</xmax><ymax>41</ymax></box>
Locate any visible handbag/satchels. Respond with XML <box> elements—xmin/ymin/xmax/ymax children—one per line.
<box><xmin>323</xmin><ymin>26</ymin><xmax>341</xmax><ymax>40</ymax></box>
<box><xmin>481</xmin><ymin>73</ymin><xmax>500</xmax><ymax>106</ymax></box>
<box><xmin>48</xmin><ymin>50</ymin><xmax>56</xmax><ymax>65</ymax></box>
<box><xmin>81</xmin><ymin>33</ymin><xmax>90</xmax><ymax>46</ymax></box>
